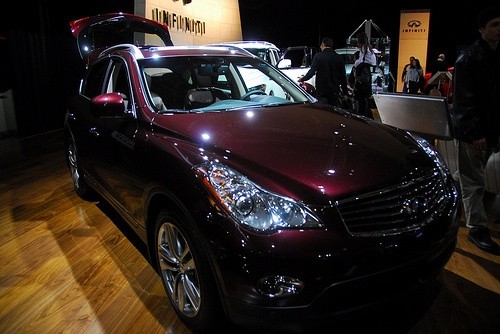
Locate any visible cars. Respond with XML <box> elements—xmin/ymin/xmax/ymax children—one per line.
<box><xmin>209</xmin><ymin>40</ymin><xmax>316</xmax><ymax>103</ymax></box>
<box><xmin>336</xmin><ymin>47</ymin><xmax>385</xmax><ymax>93</ymax></box>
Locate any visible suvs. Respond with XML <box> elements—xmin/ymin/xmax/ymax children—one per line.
<box><xmin>66</xmin><ymin>12</ymin><xmax>460</xmax><ymax>328</ymax></box>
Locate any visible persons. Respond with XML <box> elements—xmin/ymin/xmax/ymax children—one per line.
<box><xmin>297</xmin><ymin>38</ymin><xmax>348</xmax><ymax>107</ymax></box>
<box><xmin>353</xmin><ymin>33</ymin><xmax>377</xmax><ymax>70</ymax></box>
<box><xmin>452</xmin><ymin>15</ymin><xmax>500</xmax><ymax>252</ymax></box>
<box><xmin>431</xmin><ymin>54</ymin><xmax>448</xmax><ymax>96</ymax></box>
<box><xmin>348</xmin><ymin>51</ymin><xmax>374</xmax><ymax>121</ymax></box>
<box><xmin>401</xmin><ymin>57</ymin><xmax>424</xmax><ymax>94</ymax></box>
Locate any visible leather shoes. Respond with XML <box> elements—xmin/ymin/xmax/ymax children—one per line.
<box><xmin>468</xmin><ymin>228</ymin><xmax>500</xmax><ymax>256</ymax></box>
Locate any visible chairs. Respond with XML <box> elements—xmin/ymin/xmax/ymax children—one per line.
<box><xmin>146</xmin><ymin>75</ymin><xmax>167</xmax><ymax>111</ymax></box>
<box><xmin>183</xmin><ymin>67</ymin><xmax>230</xmax><ymax>110</ymax></box>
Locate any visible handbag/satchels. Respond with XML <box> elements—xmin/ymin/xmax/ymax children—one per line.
<box><xmin>418</xmin><ymin>76</ymin><xmax>425</xmax><ymax>88</ymax></box>
<box><xmin>354</xmin><ymin>62</ymin><xmax>372</xmax><ymax>92</ymax></box>
